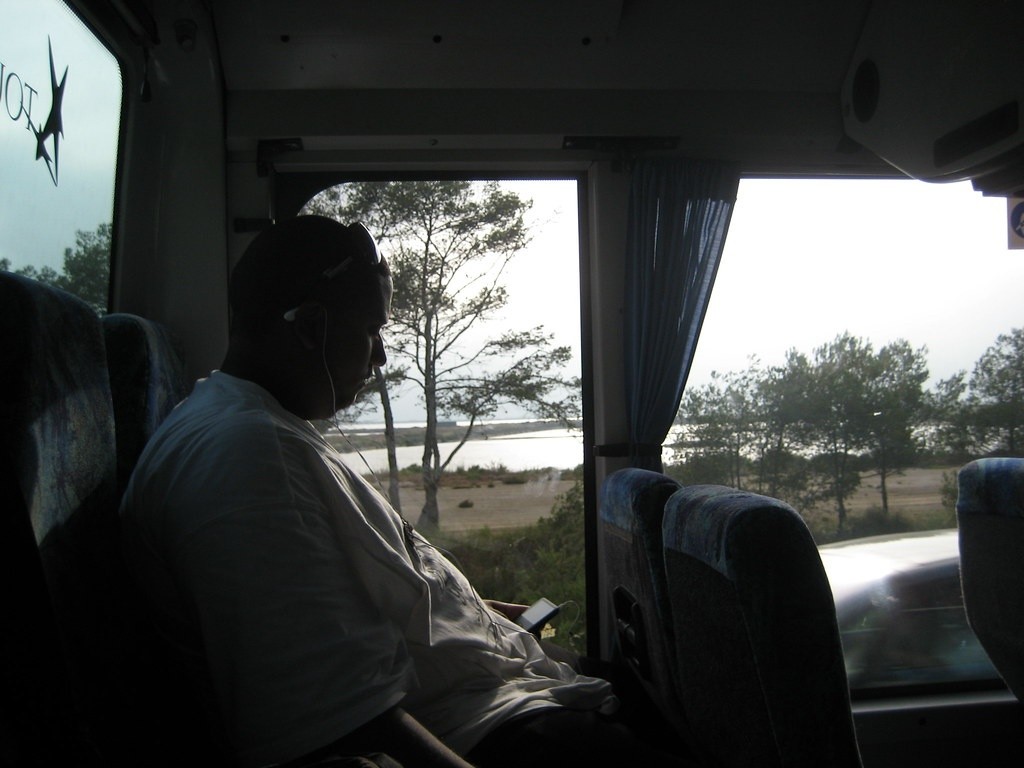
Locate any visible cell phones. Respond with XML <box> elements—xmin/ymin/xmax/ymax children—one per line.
<box><xmin>513</xmin><ymin>597</ymin><xmax>560</xmax><ymax>632</ymax></box>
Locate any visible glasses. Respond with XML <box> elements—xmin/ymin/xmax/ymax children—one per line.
<box><xmin>293</xmin><ymin>221</ymin><xmax>379</xmax><ymax>316</ymax></box>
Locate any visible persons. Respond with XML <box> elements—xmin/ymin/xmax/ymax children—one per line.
<box><xmin>116</xmin><ymin>214</ymin><xmax>655</xmax><ymax>768</ymax></box>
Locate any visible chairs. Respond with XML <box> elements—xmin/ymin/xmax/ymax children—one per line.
<box><xmin>0</xmin><ymin>270</ymin><xmax>192</xmax><ymax>768</ymax></box>
<box><xmin>956</xmin><ymin>457</ymin><xmax>1024</xmax><ymax>707</ymax></box>
<box><xmin>599</xmin><ymin>468</ymin><xmax>864</xmax><ymax>768</ymax></box>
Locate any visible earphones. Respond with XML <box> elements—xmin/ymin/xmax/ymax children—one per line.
<box><xmin>284</xmin><ymin>308</ymin><xmax>300</xmax><ymax>321</ymax></box>
